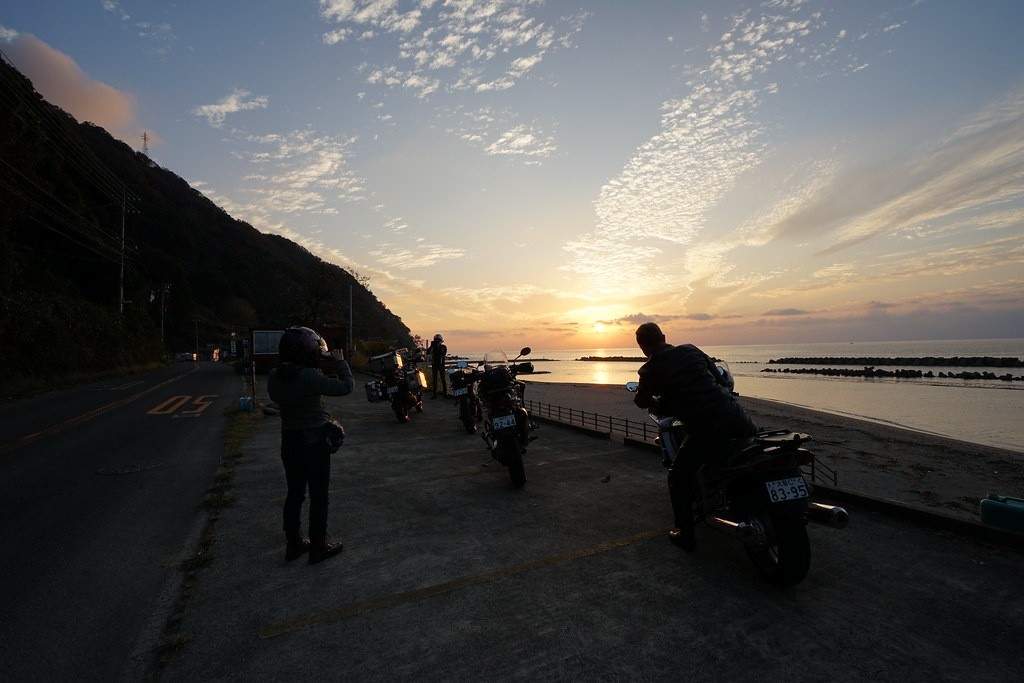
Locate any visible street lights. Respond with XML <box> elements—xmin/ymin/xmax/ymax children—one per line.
<box><xmin>348</xmin><ymin>280</ymin><xmax>353</xmax><ymax>363</ymax></box>
<box><xmin>160</xmin><ymin>289</ymin><xmax>170</xmax><ymax>352</ymax></box>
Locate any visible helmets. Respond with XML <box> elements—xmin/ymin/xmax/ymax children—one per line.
<box><xmin>434</xmin><ymin>334</ymin><xmax>443</xmax><ymax>344</ymax></box>
<box><xmin>278</xmin><ymin>325</ymin><xmax>329</xmax><ymax>366</ymax></box>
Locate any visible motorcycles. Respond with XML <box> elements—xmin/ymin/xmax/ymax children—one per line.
<box><xmin>627</xmin><ymin>365</ymin><xmax>850</xmax><ymax>580</ymax></box>
<box><xmin>448</xmin><ymin>347</ymin><xmax>540</xmax><ymax>486</ymax></box>
<box><xmin>364</xmin><ymin>348</ymin><xmax>429</xmax><ymax>422</ymax></box>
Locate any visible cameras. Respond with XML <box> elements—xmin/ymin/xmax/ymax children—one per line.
<box><xmin>320</xmin><ymin>352</ymin><xmax>334</xmax><ymax>361</ymax></box>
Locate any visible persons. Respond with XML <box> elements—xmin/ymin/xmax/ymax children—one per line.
<box><xmin>426</xmin><ymin>335</ymin><xmax>449</xmax><ymax>400</ymax></box>
<box><xmin>633</xmin><ymin>324</ymin><xmax>760</xmax><ymax>548</ymax></box>
<box><xmin>266</xmin><ymin>326</ymin><xmax>355</xmax><ymax>564</ymax></box>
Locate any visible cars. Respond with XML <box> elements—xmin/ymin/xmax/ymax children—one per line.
<box><xmin>175</xmin><ymin>353</ymin><xmax>193</xmax><ymax>360</ymax></box>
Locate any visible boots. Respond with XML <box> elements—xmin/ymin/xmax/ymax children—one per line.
<box><xmin>308</xmin><ymin>535</ymin><xmax>343</xmax><ymax>564</ymax></box>
<box><xmin>285</xmin><ymin>529</ymin><xmax>311</xmax><ymax>561</ymax></box>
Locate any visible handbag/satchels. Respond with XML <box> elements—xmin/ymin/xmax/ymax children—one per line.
<box><xmin>324</xmin><ymin>420</ymin><xmax>346</xmax><ymax>453</ymax></box>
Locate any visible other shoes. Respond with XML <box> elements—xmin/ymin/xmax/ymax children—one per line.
<box><xmin>430</xmin><ymin>395</ymin><xmax>436</xmax><ymax>399</ymax></box>
<box><xmin>670</xmin><ymin>530</ymin><xmax>695</xmax><ymax>552</ymax></box>
